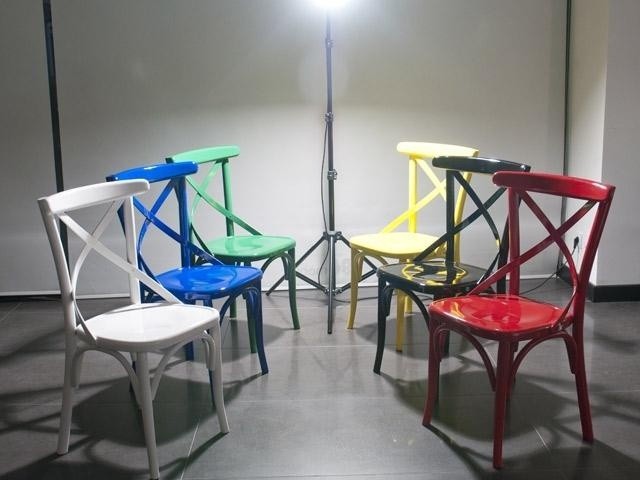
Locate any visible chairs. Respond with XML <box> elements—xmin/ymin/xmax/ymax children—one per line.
<box><xmin>165</xmin><ymin>145</ymin><xmax>301</xmax><ymax>354</ymax></box>
<box><xmin>36</xmin><ymin>177</ymin><xmax>232</xmax><ymax>480</ymax></box>
<box><xmin>373</xmin><ymin>155</ymin><xmax>531</xmax><ymax>410</ymax></box>
<box><xmin>420</xmin><ymin>170</ymin><xmax>617</xmax><ymax>470</ymax></box>
<box><xmin>105</xmin><ymin>162</ymin><xmax>268</xmax><ymax>407</ymax></box>
<box><xmin>346</xmin><ymin>141</ymin><xmax>479</xmax><ymax>353</ymax></box>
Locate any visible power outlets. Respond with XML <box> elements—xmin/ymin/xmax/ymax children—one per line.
<box><xmin>574</xmin><ymin>235</ymin><xmax>582</xmax><ymax>249</ymax></box>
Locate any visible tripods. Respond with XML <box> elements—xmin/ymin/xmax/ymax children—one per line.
<box><xmin>264</xmin><ymin>26</ymin><xmax>377</xmax><ymax>337</ymax></box>
